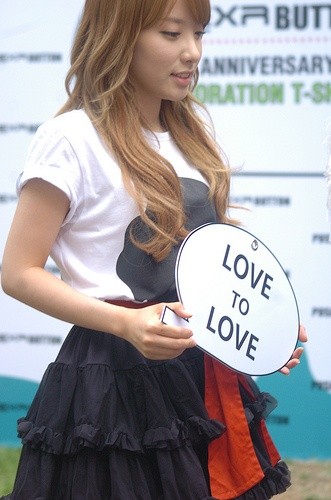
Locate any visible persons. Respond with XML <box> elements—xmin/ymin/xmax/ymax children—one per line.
<box><xmin>0</xmin><ymin>2</ymin><xmax>309</xmax><ymax>499</ymax></box>
